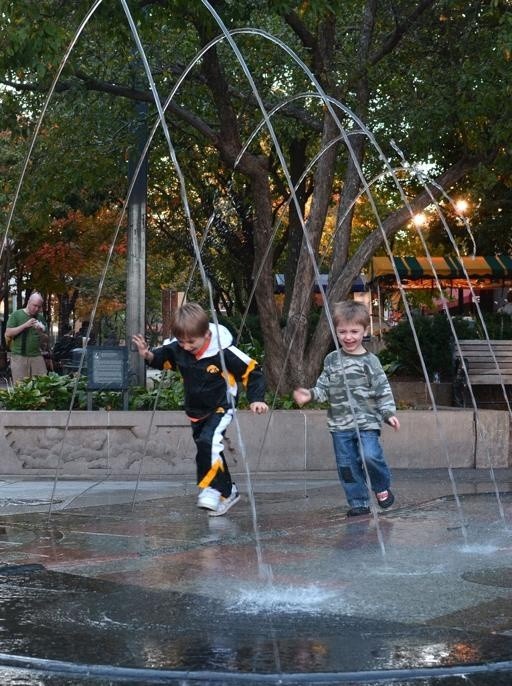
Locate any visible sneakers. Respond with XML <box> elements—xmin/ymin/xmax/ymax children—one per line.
<box><xmin>347</xmin><ymin>506</ymin><xmax>371</xmax><ymax>516</ymax></box>
<box><xmin>196</xmin><ymin>487</ymin><xmax>221</xmax><ymax>510</ymax></box>
<box><xmin>376</xmin><ymin>490</ymin><xmax>394</xmax><ymax>507</ymax></box>
<box><xmin>207</xmin><ymin>483</ymin><xmax>241</xmax><ymax>516</ymax></box>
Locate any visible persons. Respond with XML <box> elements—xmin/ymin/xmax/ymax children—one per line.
<box><xmin>292</xmin><ymin>299</ymin><xmax>400</xmax><ymax>517</ymax></box>
<box><xmin>132</xmin><ymin>300</ymin><xmax>270</xmax><ymax>516</ymax></box>
<box><xmin>4</xmin><ymin>292</ymin><xmax>48</xmax><ymax>384</ymax></box>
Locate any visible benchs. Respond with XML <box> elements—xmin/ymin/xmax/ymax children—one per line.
<box><xmin>450</xmin><ymin>331</ymin><xmax>512</xmax><ymax>410</ymax></box>
